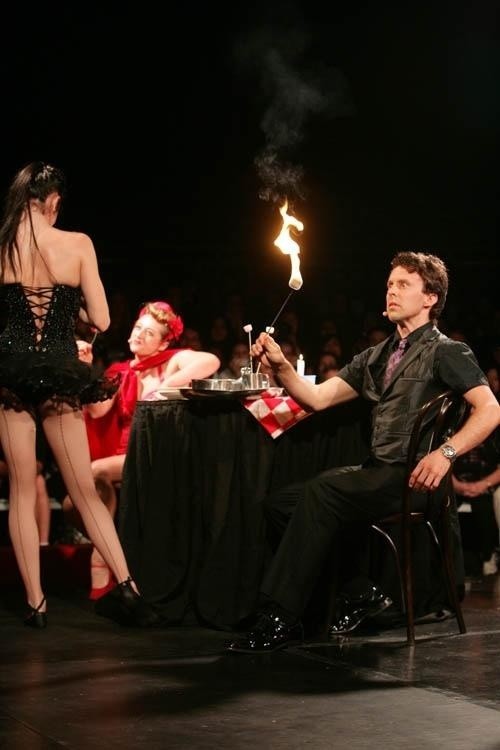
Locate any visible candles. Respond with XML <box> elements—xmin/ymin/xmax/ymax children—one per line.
<box><xmin>296</xmin><ymin>354</ymin><xmax>305</xmax><ymax>376</ymax></box>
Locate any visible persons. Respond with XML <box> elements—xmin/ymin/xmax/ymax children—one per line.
<box><xmin>2</xmin><ymin>161</ymin><xmax>158</xmax><ymax>634</ymax></box>
<box><xmin>1</xmin><ymin>286</ymin><xmax>500</xmax><ymax>600</ymax></box>
<box><xmin>225</xmin><ymin>249</ymin><xmax>500</xmax><ymax>654</ymax></box>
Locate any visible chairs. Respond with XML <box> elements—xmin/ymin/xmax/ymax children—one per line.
<box><xmin>322</xmin><ymin>389</ymin><xmax>466</xmax><ymax>647</ymax></box>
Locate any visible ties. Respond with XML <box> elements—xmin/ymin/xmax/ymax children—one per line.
<box><xmin>382</xmin><ymin>338</ymin><xmax>407</xmax><ymax>393</ymax></box>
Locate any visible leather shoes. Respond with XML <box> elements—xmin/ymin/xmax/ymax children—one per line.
<box><xmin>89</xmin><ymin>562</ymin><xmax>116</xmax><ymax>600</ymax></box>
<box><xmin>224</xmin><ymin>611</ymin><xmax>305</xmax><ymax>654</ymax></box>
<box><xmin>328</xmin><ymin>585</ymin><xmax>393</xmax><ymax>634</ymax></box>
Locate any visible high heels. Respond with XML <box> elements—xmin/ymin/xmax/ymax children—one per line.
<box><xmin>24</xmin><ymin>597</ymin><xmax>49</xmax><ymax>628</ymax></box>
<box><xmin>119</xmin><ymin>576</ymin><xmax>159</xmax><ymax>628</ymax></box>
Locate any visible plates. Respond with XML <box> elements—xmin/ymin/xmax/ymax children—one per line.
<box><xmin>159</xmin><ymin>390</ymin><xmax>183</xmax><ymax>400</ymax></box>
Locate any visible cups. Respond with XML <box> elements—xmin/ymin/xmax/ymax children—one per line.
<box><xmin>301</xmin><ymin>375</ymin><xmax>316</xmax><ymax>385</ymax></box>
<box><xmin>192</xmin><ymin>373</ymin><xmax>269</xmax><ymax>389</ymax></box>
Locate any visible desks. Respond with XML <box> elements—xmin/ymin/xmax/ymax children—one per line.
<box><xmin>94</xmin><ymin>395</ymin><xmax>372</xmax><ymax>633</ymax></box>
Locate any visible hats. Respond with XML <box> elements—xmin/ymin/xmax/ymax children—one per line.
<box><xmin>139</xmin><ymin>301</ymin><xmax>184</xmax><ymax>342</ymax></box>
